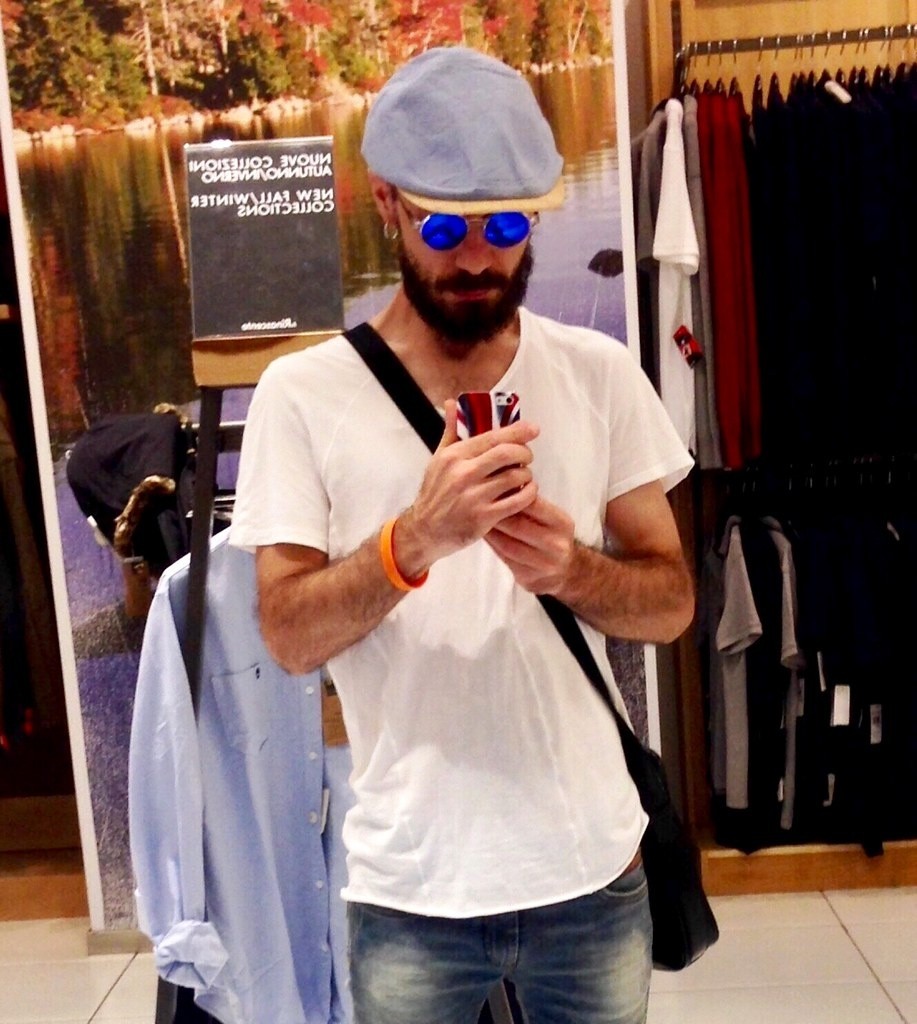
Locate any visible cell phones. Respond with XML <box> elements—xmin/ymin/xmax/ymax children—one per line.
<box><xmin>456</xmin><ymin>393</ymin><xmax>522</xmax><ymax>501</ymax></box>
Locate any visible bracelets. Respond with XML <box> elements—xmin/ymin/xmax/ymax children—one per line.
<box><xmin>380</xmin><ymin>519</ymin><xmax>428</xmax><ymax>591</ymax></box>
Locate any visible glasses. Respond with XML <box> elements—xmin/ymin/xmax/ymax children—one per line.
<box><xmin>395</xmin><ymin>194</ymin><xmax>539</xmax><ymax>249</ymax></box>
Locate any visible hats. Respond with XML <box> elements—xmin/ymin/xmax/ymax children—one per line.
<box><xmin>360</xmin><ymin>47</ymin><xmax>564</xmax><ymax>215</ymax></box>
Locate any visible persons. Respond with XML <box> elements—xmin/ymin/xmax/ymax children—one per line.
<box><xmin>226</xmin><ymin>47</ymin><xmax>696</xmax><ymax>1024</ymax></box>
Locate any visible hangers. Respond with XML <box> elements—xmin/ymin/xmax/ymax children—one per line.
<box><xmin>652</xmin><ymin>21</ymin><xmax>917</xmax><ymax>119</ymax></box>
<box><xmin>718</xmin><ymin>454</ymin><xmax>916</xmax><ymax>515</ymax></box>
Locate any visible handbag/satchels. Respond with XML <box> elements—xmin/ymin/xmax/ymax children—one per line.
<box><xmin>622</xmin><ymin>734</ymin><xmax>719</xmax><ymax>972</ymax></box>
